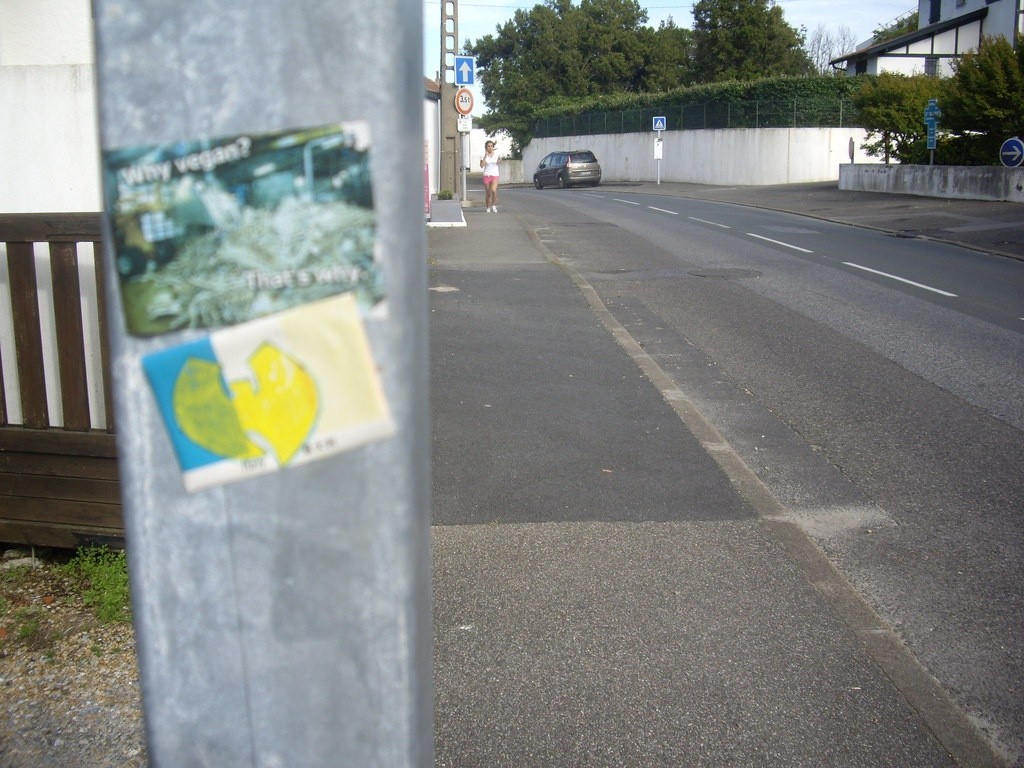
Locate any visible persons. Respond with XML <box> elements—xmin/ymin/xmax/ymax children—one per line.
<box><xmin>480</xmin><ymin>140</ymin><xmax>502</xmax><ymax>213</ymax></box>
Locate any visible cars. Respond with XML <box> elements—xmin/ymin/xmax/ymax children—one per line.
<box><xmin>533</xmin><ymin>149</ymin><xmax>602</xmax><ymax>190</ymax></box>
